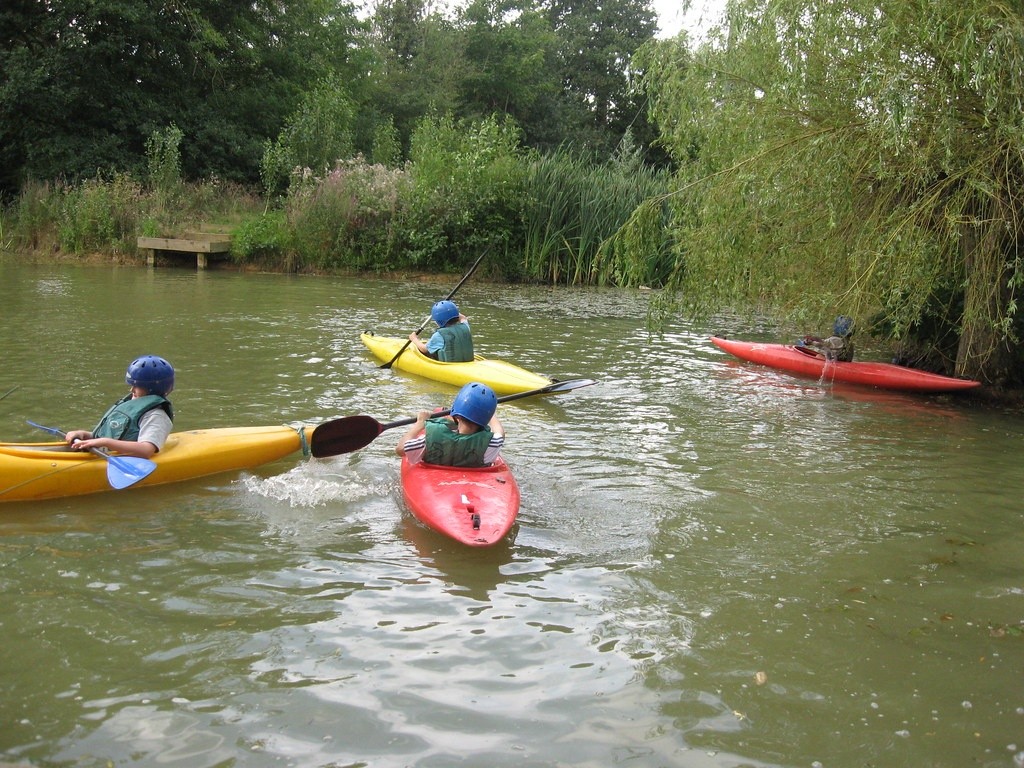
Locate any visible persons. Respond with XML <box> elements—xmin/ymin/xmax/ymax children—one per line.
<box><xmin>65</xmin><ymin>356</ymin><xmax>173</xmax><ymax>459</ymax></box>
<box><xmin>395</xmin><ymin>383</ymin><xmax>505</xmax><ymax>467</ymax></box>
<box><xmin>409</xmin><ymin>301</ymin><xmax>474</xmax><ymax>362</ymax></box>
<box><xmin>803</xmin><ymin>315</ymin><xmax>856</xmax><ymax>362</ymax></box>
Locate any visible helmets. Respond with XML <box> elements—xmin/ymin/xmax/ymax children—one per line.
<box><xmin>125</xmin><ymin>355</ymin><xmax>174</xmax><ymax>396</ymax></box>
<box><xmin>450</xmin><ymin>382</ymin><xmax>498</xmax><ymax>427</ymax></box>
<box><xmin>834</xmin><ymin>315</ymin><xmax>855</xmax><ymax>336</ymax></box>
<box><xmin>430</xmin><ymin>301</ymin><xmax>459</xmax><ymax>328</ymax></box>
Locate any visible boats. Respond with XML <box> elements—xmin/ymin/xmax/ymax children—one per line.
<box><xmin>401</xmin><ymin>407</ymin><xmax>521</xmax><ymax>548</ymax></box>
<box><xmin>708</xmin><ymin>333</ymin><xmax>983</xmax><ymax>392</ymax></box>
<box><xmin>0</xmin><ymin>426</ymin><xmax>318</xmax><ymax>505</ymax></box>
<box><xmin>361</xmin><ymin>329</ymin><xmax>572</xmax><ymax>398</ymax></box>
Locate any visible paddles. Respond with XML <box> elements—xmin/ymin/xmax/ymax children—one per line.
<box><xmin>378</xmin><ymin>242</ymin><xmax>494</xmax><ymax>369</ymax></box>
<box><xmin>310</xmin><ymin>378</ymin><xmax>600</xmax><ymax>458</ymax></box>
<box><xmin>25</xmin><ymin>419</ymin><xmax>159</xmax><ymax>491</ymax></box>
